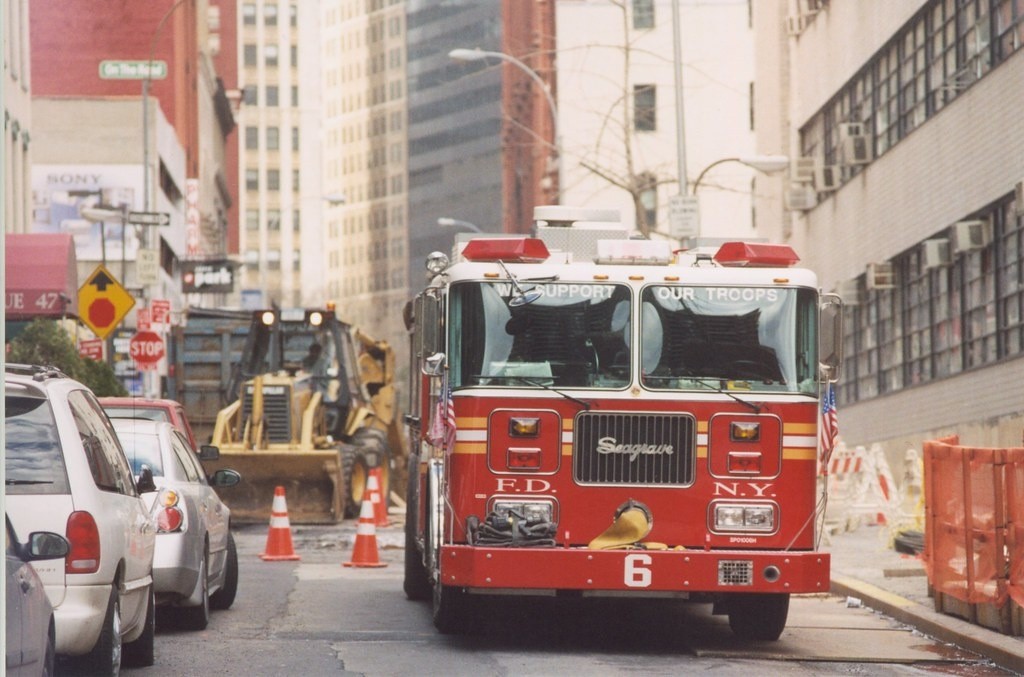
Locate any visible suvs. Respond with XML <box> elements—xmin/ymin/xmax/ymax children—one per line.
<box><xmin>5</xmin><ymin>360</ymin><xmax>159</xmax><ymax>677</ymax></box>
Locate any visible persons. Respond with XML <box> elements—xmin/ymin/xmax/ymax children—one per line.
<box><xmin>302</xmin><ymin>344</ymin><xmax>330</xmax><ymax>377</ymax></box>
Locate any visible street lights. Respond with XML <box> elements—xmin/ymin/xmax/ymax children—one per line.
<box><xmin>447</xmin><ymin>49</ymin><xmax>567</xmax><ymax>204</ymax></box>
<box><xmin>666</xmin><ymin>2</ymin><xmax>790</xmax><ymax>249</ymax></box>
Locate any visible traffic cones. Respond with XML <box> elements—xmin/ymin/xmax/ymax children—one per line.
<box><xmin>353</xmin><ymin>467</ymin><xmax>400</xmax><ymax>527</ymax></box>
<box><xmin>256</xmin><ymin>484</ymin><xmax>301</xmax><ymax>561</ymax></box>
<box><xmin>340</xmin><ymin>489</ymin><xmax>389</xmax><ymax>569</ymax></box>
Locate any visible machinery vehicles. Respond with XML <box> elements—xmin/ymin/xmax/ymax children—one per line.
<box><xmin>195</xmin><ymin>302</ymin><xmax>412</xmax><ymax>528</ymax></box>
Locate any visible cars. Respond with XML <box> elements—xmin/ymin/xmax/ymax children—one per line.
<box><xmin>5</xmin><ymin>509</ymin><xmax>72</xmax><ymax>677</ymax></box>
<box><xmin>106</xmin><ymin>417</ymin><xmax>242</xmax><ymax>629</ymax></box>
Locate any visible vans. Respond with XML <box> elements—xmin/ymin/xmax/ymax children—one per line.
<box><xmin>92</xmin><ymin>395</ymin><xmax>220</xmax><ymax>463</ymax></box>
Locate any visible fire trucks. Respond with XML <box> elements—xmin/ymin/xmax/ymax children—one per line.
<box><xmin>398</xmin><ymin>202</ymin><xmax>845</xmax><ymax>644</ymax></box>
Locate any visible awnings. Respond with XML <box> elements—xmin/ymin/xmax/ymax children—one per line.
<box><xmin>4</xmin><ymin>234</ymin><xmax>79</xmax><ymax>322</ymax></box>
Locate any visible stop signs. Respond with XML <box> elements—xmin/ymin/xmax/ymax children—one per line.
<box><xmin>128</xmin><ymin>330</ymin><xmax>166</xmax><ymax>365</ymax></box>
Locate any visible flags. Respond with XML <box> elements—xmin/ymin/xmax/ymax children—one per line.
<box><xmin>820</xmin><ymin>382</ymin><xmax>838</xmax><ymax>474</ymax></box>
